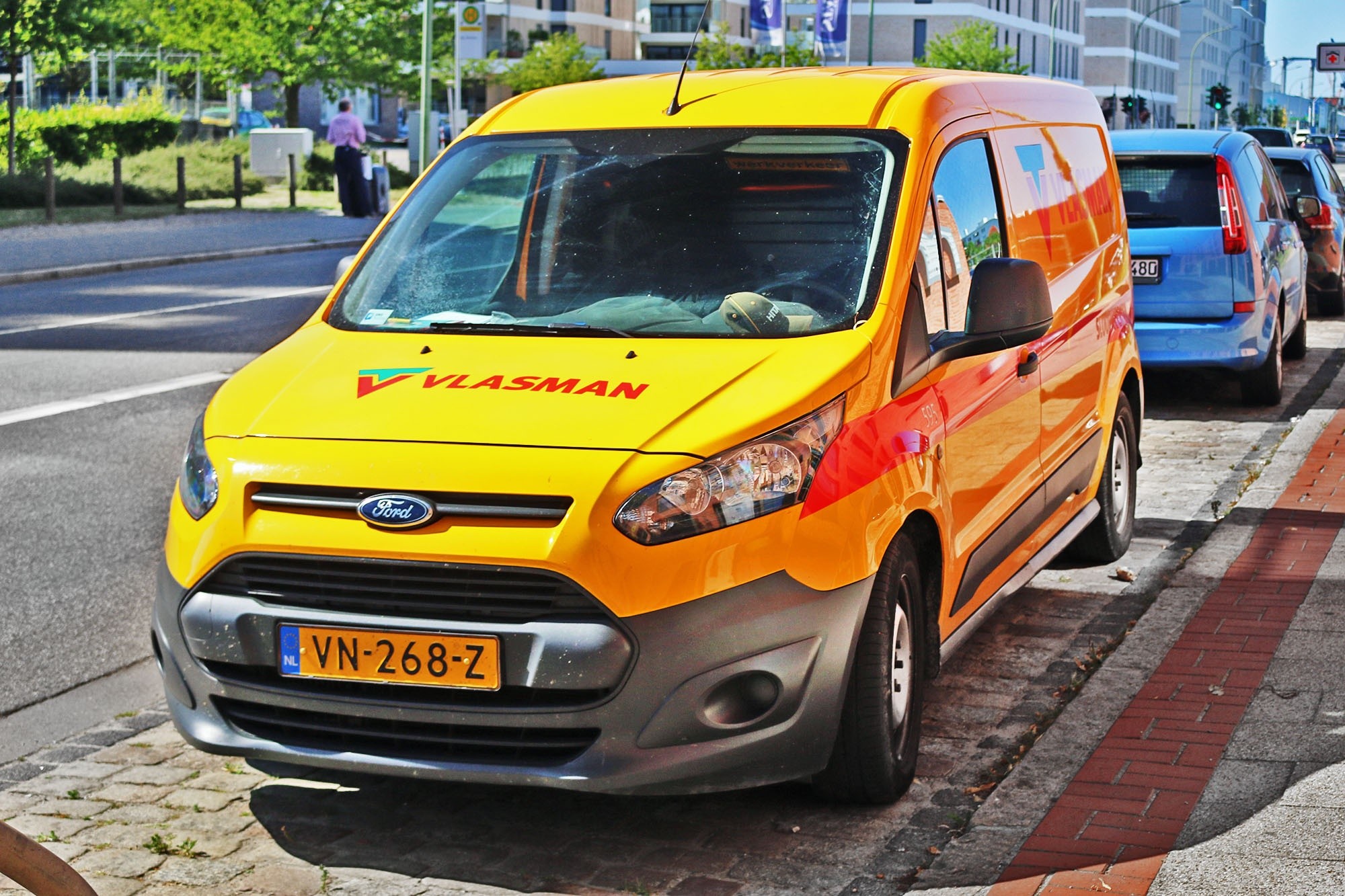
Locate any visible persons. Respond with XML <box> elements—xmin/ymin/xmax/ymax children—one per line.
<box><xmin>325</xmin><ymin>99</ymin><xmax>378</xmax><ymax>217</ymax></box>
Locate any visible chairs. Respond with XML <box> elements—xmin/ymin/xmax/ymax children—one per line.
<box><xmin>564</xmin><ymin>157</ymin><xmax>753</xmax><ymax>300</ymax></box>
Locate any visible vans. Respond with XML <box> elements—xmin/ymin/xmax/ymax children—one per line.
<box><xmin>146</xmin><ymin>66</ymin><xmax>1146</xmax><ymax>812</ymax></box>
<box><xmin>1241</xmin><ymin>126</ymin><xmax>1294</xmax><ymax>147</ymax></box>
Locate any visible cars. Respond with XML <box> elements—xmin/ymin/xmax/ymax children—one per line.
<box><xmin>1104</xmin><ymin>129</ymin><xmax>1322</xmax><ymax>407</ymax></box>
<box><xmin>1294</xmin><ymin>129</ymin><xmax>1345</xmax><ymax>161</ymax></box>
<box><xmin>1260</xmin><ymin>145</ymin><xmax>1345</xmax><ymax>318</ymax></box>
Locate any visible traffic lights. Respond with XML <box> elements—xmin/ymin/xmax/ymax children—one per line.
<box><xmin>1205</xmin><ymin>86</ymin><xmax>1233</xmax><ymax>110</ymax></box>
<box><xmin>1120</xmin><ymin>95</ymin><xmax>1147</xmax><ymax>114</ymax></box>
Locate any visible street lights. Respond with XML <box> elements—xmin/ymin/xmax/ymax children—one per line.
<box><xmin>1130</xmin><ymin>0</ymin><xmax>1193</xmax><ymax>128</ymax></box>
<box><xmin>1222</xmin><ymin>39</ymin><xmax>1264</xmax><ymax>87</ymax></box>
<box><xmin>1186</xmin><ymin>24</ymin><xmax>1239</xmax><ymax>129</ymax></box>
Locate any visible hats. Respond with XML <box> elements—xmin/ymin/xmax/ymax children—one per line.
<box><xmin>719</xmin><ymin>291</ymin><xmax>813</xmax><ymax>335</ymax></box>
